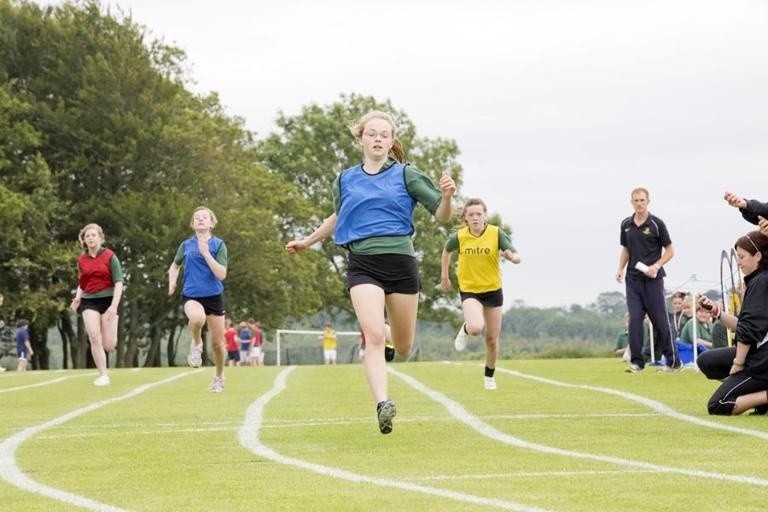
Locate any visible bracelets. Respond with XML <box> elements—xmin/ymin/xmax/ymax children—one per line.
<box><xmin>655</xmin><ymin>263</ymin><xmax>660</xmax><ymax>269</ymax></box>
<box><xmin>733</xmin><ymin>358</ymin><xmax>743</xmax><ymax>367</ymax></box>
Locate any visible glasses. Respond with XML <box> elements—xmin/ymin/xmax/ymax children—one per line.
<box><xmin>361</xmin><ymin>130</ymin><xmax>390</xmax><ymax>139</ymax></box>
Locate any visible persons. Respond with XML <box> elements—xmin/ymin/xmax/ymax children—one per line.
<box><xmin>285</xmin><ymin>111</ymin><xmax>458</xmax><ymax>434</ymax></box>
<box><xmin>70</xmin><ymin>223</ymin><xmax>126</xmax><ymax>387</ymax></box>
<box><xmin>440</xmin><ymin>199</ymin><xmax>521</xmax><ymax>389</ymax></box>
<box><xmin>356</xmin><ymin>330</ymin><xmax>365</xmax><ymax>364</ymax></box>
<box><xmin>696</xmin><ymin>232</ymin><xmax>768</xmax><ymax>415</ymax></box>
<box><xmin>757</xmin><ymin>215</ymin><xmax>768</xmax><ymax>236</ymax></box>
<box><xmin>167</xmin><ymin>207</ymin><xmax>228</xmax><ymax>393</ymax></box>
<box><xmin>14</xmin><ymin>318</ymin><xmax>35</xmax><ymax>371</ymax></box>
<box><xmin>723</xmin><ymin>191</ymin><xmax>768</xmax><ymax>232</ymax></box>
<box><xmin>615</xmin><ymin>292</ymin><xmax>727</xmax><ymax>369</ymax></box>
<box><xmin>224</xmin><ymin>317</ymin><xmax>265</xmax><ymax>366</ymax></box>
<box><xmin>0</xmin><ymin>294</ymin><xmax>7</xmax><ymax>372</ymax></box>
<box><xmin>318</xmin><ymin>323</ymin><xmax>337</xmax><ymax>365</ymax></box>
<box><xmin>616</xmin><ymin>188</ymin><xmax>686</xmax><ymax>373</ymax></box>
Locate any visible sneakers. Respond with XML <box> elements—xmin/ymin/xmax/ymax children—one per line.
<box><xmin>378</xmin><ymin>402</ymin><xmax>396</xmax><ymax>434</ymax></box>
<box><xmin>211</xmin><ymin>372</ymin><xmax>225</xmax><ymax>393</ymax></box>
<box><xmin>455</xmin><ymin>320</ymin><xmax>469</xmax><ymax>352</ymax></box>
<box><xmin>663</xmin><ymin>365</ymin><xmax>685</xmax><ymax>374</ymax></box>
<box><xmin>188</xmin><ymin>339</ymin><xmax>203</xmax><ymax>367</ymax></box>
<box><xmin>385</xmin><ymin>321</ymin><xmax>394</xmax><ymax>362</ymax></box>
<box><xmin>484</xmin><ymin>375</ymin><xmax>496</xmax><ymax>390</ymax></box>
<box><xmin>625</xmin><ymin>364</ymin><xmax>642</xmax><ymax>372</ymax></box>
<box><xmin>95</xmin><ymin>376</ymin><xmax>109</xmax><ymax>385</ymax></box>
